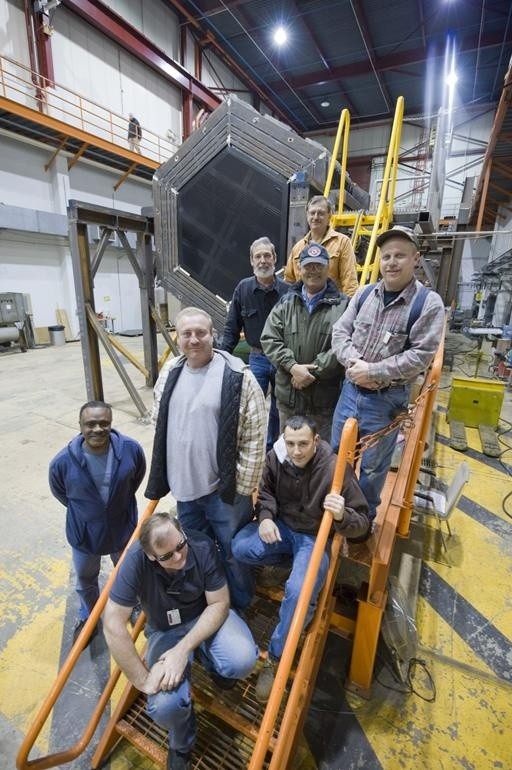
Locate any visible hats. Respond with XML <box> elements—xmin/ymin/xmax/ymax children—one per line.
<box><xmin>300</xmin><ymin>242</ymin><xmax>329</xmax><ymax>267</ymax></box>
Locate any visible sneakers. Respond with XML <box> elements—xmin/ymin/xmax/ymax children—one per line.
<box><xmin>255</xmin><ymin>657</ymin><xmax>279</xmax><ymax>704</ymax></box>
<box><xmin>208</xmin><ymin>672</ymin><xmax>237</xmax><ymax>690</ymax></box>
<box><xmin>376</xmin><ymin>225</ymin><xmax>420</xmax><ymax>250</ymax></box>
<box><xmin>167</xmin><ymin>747</ymin><xmax>192</xmax><ymax>770</ymax></box>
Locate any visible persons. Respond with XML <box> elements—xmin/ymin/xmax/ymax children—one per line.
<box><xmin>329</xmin><ymin>225</ymin><xmax>445</xmax><ymax>545</ymax></box>
<box><xmin>143</xmin><ymin>306</ymin><xmax>270</xmax><ymax>570</ymax></box>
<box><xmin>127</xmin><ymin>112</ymin><xmax>144</xmax><ymax>156</ymax></box>
<box><xmin>218</xmin><ymin>236</ymin><xmax>292</xmax><ymax>451</ymax></box>
<box><xmin>96</xmin><ymin>510</ymin><xmax>261</xmax><ymax>770</ymax></box>
<box><xmin>228</xmin><ymin>414</ymin><xmax>374</xmax><ymax>706</ymax></box>
<box><xmin>283</xmin><ymin>195</ymin><xmax>359</xmax><ymax>301</ymax></box>
<box><xmin>260</xmin><ymin>243</ymin><xmax>353</xmax><ymax>440</ymax></box>
<box><xmin>47</xmin><ymin>401</ymin><xmax>146</xmax><ymax>649</ymax></box>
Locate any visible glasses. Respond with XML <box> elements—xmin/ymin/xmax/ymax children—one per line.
<box><xmin>152</xmin><ymin>527</ymin><xmax>188</xmax><ymax>561</ymax></box>
<box><xmin>82</xmin><ymin>421</ymin><xmax>111</xmax><ymax>427</ymax></box>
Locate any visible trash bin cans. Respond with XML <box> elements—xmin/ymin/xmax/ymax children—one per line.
<box><xmin>48</xmin><ymin>326</ymin><xmax>65</xmax><ymax>346</ymax></box>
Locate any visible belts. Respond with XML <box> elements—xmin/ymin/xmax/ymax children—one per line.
<box><xmin>349</xmin><ymin>382</ymin><xmax>404</xmax><ymax>393</ymax></box>
